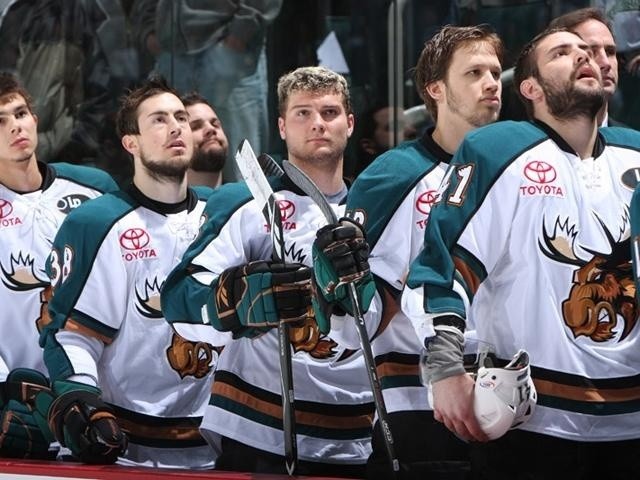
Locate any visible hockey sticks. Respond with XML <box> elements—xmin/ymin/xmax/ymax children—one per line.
<box><xmin>256</xmin><ymin>153</ymin><xmax>284</xmax><ymax>178</ymax></box>
<box><xmin>282</xmin><ymin>159</ymin><xmax>402</xmax><ymax>474</ymax></box>
<box><xmin>233</xmin><ymin>138</ymin><xmax>298</xmax><ymax>477</ymax></box>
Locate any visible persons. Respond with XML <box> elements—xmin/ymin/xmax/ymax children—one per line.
<box><xmin>176</xmin><ymin>90</ymin><xmax>230</xmax><ymax>189</ymax></box>
<box><xmin>349</xmin><ymin>94</ymin><xmax>418</xmax><ymax>166</ymax></box>
<box><xmin>401</xmin><ymin>28</ymin><xmax>640</xmax><ymax>480</ymax></box>
<box><xmin>313</xmin><ymin>27</ymin><xmax>502</xmax><ymax>476</ymax></box>
<box><xmin>160</xmin><ymin>66</ymin><xmax>376</xmax><ymax>478</ymax></box>
<box><xmin>131</xmin><ymin>0</ymin><xmax>282</xmax><ymax>183</ymax></box>
<box><xmin>0</xmin><ymin>66</ymin><xmax>121</xmax><ymax>459</ymax></box>
<box><xmin>551</xmin><ymin>8</ymin><xmax>631</xmax><ymax>129</ymax></box>
<box><xmin>40</xmin><ymin>75</ymin><xmax>225</xmax><ymax>470</ymax></box>
<box><xmin>15</xmin><ymin>0</ymin><xmax>99</xmax><ymax>162</ymax></box>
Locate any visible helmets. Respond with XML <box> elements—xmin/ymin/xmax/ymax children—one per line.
<box><xmin>419</xmin><ymin>338</ymin><xmax>538</xmax><ymax>443</ymax></box>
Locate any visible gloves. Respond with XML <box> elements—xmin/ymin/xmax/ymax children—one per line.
<box><xmin>207</xmin><ymin>260</ymin><xmax>314</xmax><ymax>340</ymax></box>
<box><xmin>312</xmin><ymin>217</ymin><xmax>376</xmax><ymax>316</ymax></box>
<box><xmin>0</xmin><ymin>368</ymin><xmax>58</xmax><ymax>459</ymax></box>
<box><xmin>33</xmin><ymin>379</ymin><xmax>129</xmax><ymax>465</ymax></box>
